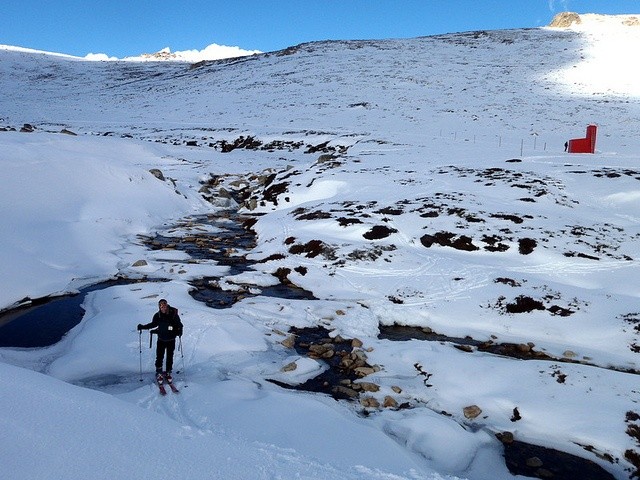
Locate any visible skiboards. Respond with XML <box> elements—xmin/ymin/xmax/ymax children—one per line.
<box><xmin>159</xmin><ymin>383</ymin><xmax>179</xmax><ymax>395</ymax></box>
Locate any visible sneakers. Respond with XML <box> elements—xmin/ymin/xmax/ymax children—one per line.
<box><xmin>165</xmin><ymin>372</ymin><xmax>172</xmax><ymax>383</ymax></box>
<box><xmin>156</xmin><ymin>373</ymin><xmax>164</xmax><ymax>385</ymax></box>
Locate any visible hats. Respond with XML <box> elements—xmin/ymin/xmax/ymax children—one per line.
<box><xmin>158</xmin><ymin>299</ymin><xmax>167</xmax><ymax>305</ymax></box>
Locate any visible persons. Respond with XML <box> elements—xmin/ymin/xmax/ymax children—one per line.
<box><xmin>137</xmin><ymin>299</ymin><xmax>184</xmax><ymax>384</ymax></box>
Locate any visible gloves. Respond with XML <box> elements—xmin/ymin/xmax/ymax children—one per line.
<box><xmin>138</xmin><ymin>324</ymin><xmax>144</xmax><ymax>330</ymax></box>
